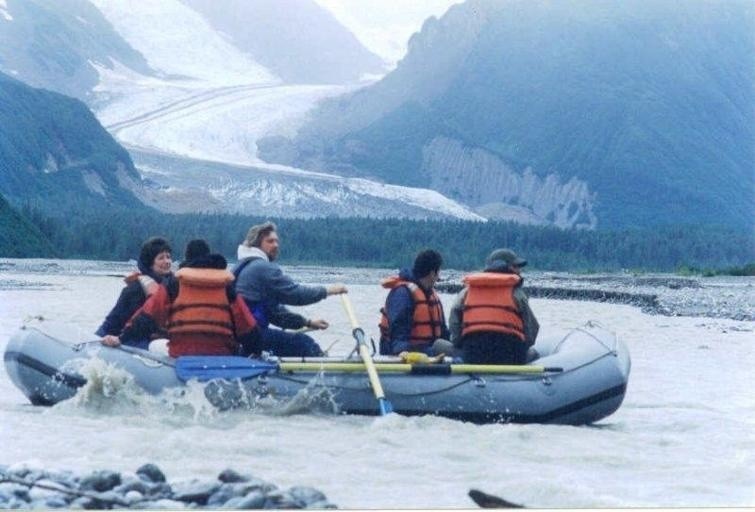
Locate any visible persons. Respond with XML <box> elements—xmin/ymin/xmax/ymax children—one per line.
<box><xmin>377</xmin><ymin>249</ymin><xmax>450</xmax><ymax>364</ymax></box>
<box><xmin>92</xmin><ymin>235</ymin><xmax>178</xmax><ymax>352</ymax></box>
<box><xmin>448</xmin><ymin>248</ymin><xmax>540</xmax><ymax>364</ymax></box>
<box><xmin>230</xmin><ymin>220</ymin><xmax>350</xmax><ymax>357</ymax></box>
<box><xmin>100</xmin><ymin>238</ymin><xmax>272</xmax><ymax>357</ymax></box>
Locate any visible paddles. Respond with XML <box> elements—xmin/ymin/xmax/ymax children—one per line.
<box><xmin>174</xmin><ymin>355</ymin><xmax>563</xmax><ymax>381</ymax></box>
<box><xmin>340</xmin><ymin>291</ymin><xmax>393</xmax><ymax>415</ymax></box>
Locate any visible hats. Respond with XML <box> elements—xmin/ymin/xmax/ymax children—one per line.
<box><xmin>245</xmin><ymin>221</ymin><xmax>277</xmax><ymax>248</ymax></box>
<box><xmin>490</xmin><ymin>249</ymin><xmax>528</xmax><ymax>268</ymax></box>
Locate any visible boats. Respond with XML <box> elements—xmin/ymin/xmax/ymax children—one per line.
<box><xmin>4</xmin><ymin>318</ymin><xmax>633</xmax><ymax>427</ymax></box>
